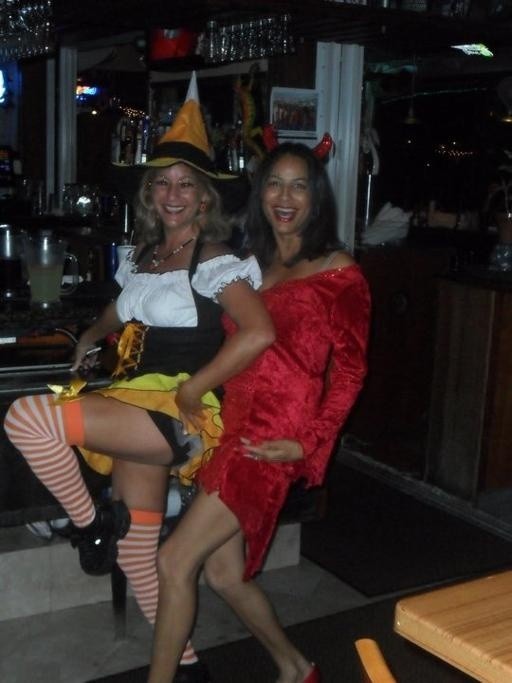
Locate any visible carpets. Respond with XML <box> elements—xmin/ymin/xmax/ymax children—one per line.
<box><xmin>85</xmin><ymin>572</ymin><xmax>512</xmax><ymax>683</ymax></box>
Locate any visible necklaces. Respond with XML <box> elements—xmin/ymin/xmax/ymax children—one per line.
<box><xmin>151</xmin><ymin>234</ymin><xmax>197</xmax><ymax>269</ymax></box>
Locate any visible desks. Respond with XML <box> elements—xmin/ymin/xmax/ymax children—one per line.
<box><xmin>393</xmin><ymin>571</ymin><xmax>512</xmax><ymax>683</ymax></box>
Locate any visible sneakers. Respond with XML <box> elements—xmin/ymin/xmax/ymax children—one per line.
<box><xmin>69</xmin><ymin>499</ymin><xmax>130</xmax><ymax>576</ymax></box>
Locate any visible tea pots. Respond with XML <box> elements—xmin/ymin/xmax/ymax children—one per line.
<box><xmin>20</xmin><ymin>235</ymin><xmax>81</xmax><ymax>302</ymax></box>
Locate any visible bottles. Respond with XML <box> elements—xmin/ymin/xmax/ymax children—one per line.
<box><xmin>273</xmin><ymin>95</ymin><xmax>318</xmax><ymax>130</ymax></box>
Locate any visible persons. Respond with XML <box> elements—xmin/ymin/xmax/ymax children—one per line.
<box><xmin>1</xmin><ymin>70</ymin><xmax>277</xmax><ymax>683</ymax></box>
<box><xmin>148</xmin><ymin>143</ymin><xmax>372</xmax><ymax>683</ymax></box>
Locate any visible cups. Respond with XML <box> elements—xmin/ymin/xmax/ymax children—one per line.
<box><xmin>204</xmin><ymin>14</ymin><xmax>292</xmax><ymax>65</ymax></box>
<box><xmin>107</xmin><ymin>241</ymin><xmax>138</xmax><ymax>282</ymax></box>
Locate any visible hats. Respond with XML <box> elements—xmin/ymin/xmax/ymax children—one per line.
<box><xmin>113</xmin><ymin>71</ymin><xmax>239</xmax><ymax>179</ymax></box>
